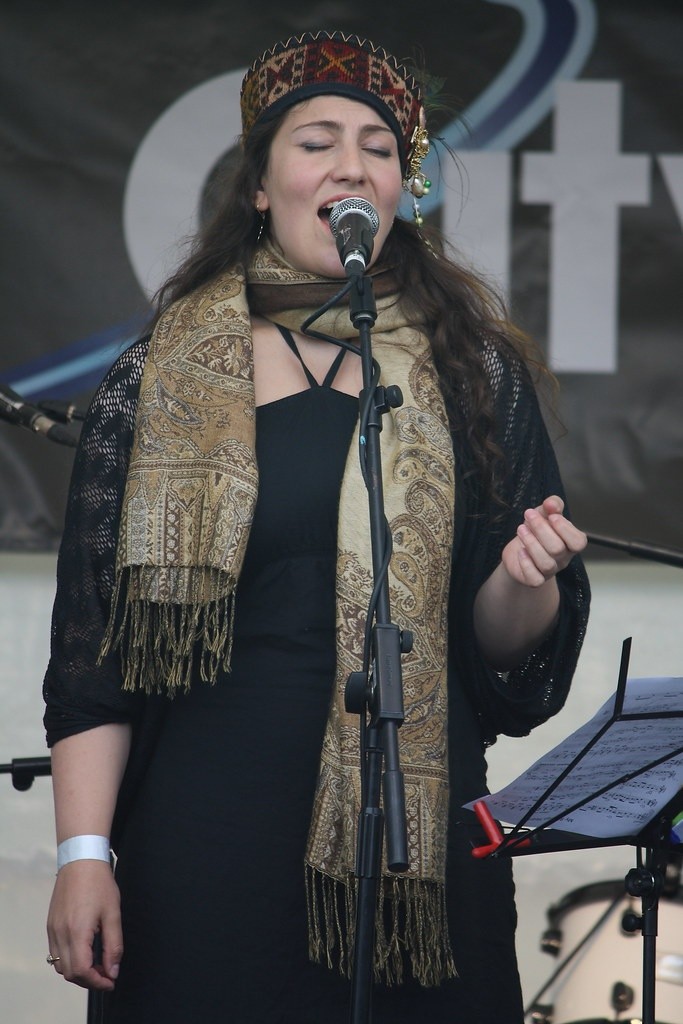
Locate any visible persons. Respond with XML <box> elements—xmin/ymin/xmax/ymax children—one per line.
<box><xmin>46</xmin><ymin>31</ymin><xmax>592</xmax><ymax>1024</ymax></box>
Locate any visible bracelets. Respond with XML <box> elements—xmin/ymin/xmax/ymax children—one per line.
<box><xmin>55</xmin><ymin>835</ymin><xmax>111</xmax><ymax>871</ymax></box>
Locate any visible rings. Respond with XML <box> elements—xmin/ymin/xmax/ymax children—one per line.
<box><xmin>47</xmin><ymin>954</ymin><xmax>60</xmax><ymax>966</ymax></box>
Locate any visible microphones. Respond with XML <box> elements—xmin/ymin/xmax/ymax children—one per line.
<box><xmin>329</xmin><ymin>196</ymin><xmax>380</xmax><ymax>281</ymax></box>
<box><xmin>0</xmin><ymin>384</ymin><xmax>79</xmax><ymax>449</ymax></box>
